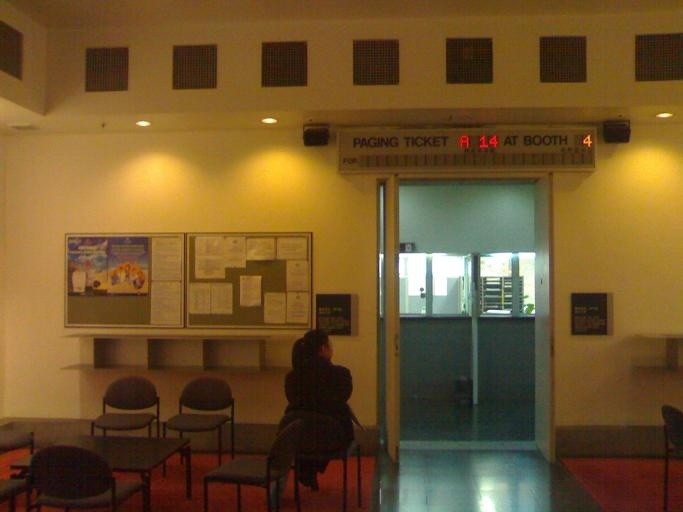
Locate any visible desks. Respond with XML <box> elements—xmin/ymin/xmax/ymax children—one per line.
<box><xmin>10</xmin><ymin>436</ymin><xmax>192</xmax><ymax>512</ymax></box>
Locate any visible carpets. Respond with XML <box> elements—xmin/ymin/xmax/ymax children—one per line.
<box><xmin>560</xmin><ymin>458</ymin><xmax>683</xmax><ymax>512</ymax></box>
<box><xmin>0</xmin><ymin>448</ymin><xmax>375</xmax><ymax>512</ymax></box>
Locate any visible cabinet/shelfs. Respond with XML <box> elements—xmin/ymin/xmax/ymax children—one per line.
<box><xmin>61</xmin><ymin>332</ymin><xmax>300</xmax><ymax>376</ymax></box>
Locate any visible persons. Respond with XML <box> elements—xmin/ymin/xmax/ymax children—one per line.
<box><xmin>275</xmin><ymin>326</ymin><xmax>366</xmax><ymax>492</ymax></box>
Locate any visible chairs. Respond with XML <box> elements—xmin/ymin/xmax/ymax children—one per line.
<box><xmin>0</xmin><ymin>430</ymin><xmax>34</xmax><ymax>454</ymax></box>
<box><xmin>204</xmin><ymin>420</ymin><xmax>304</xmax><ymax>512</ymax></box>
<box><xmin>294</xmin><ymin>438</ymin><xmax>361</xmax><ymax>512</ymax></box>
<box><xmin>662</xmin><ymin>405</ymin><xmax>683</xmax><ymax>512</ymax></box>
<box><xmin>163</xmin><ymin>378</ymin><xmax>235</xmax><ymax>475</ymax></box>
<box><xmin>26</xmin><ymin>445</ymin><xmax>147</xmax><ymax>512</ymax></box>
<box><xmin>0</xmin><ymin>478</ymin><xmax>26</xmax><ymax>511</ymax></box>
<box><xmin>91</xmin><ymin>377</ymin><xmax>160</xmax><ymax>438</ymax></box>
